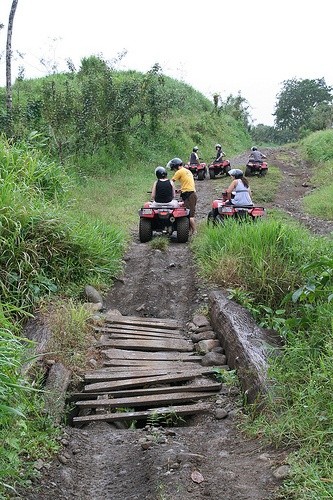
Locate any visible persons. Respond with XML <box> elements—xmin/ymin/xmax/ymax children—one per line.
<box><xmin>167</xmin><ymin>158</ymin><xmax>198</xmax><ymax>237</ymax></box>
<box><xmin>150</xmin><ymin>166</ymin><xmax>175</xmax><ymax>203</ymax></box>
<box><xmin>249</xmin><ymin>146</ymin><xmax>266</xmax><ymax>164</ymax></box>
<box><xmin>226</xmin><ymin>169</ymin><xmax>254</xmax><ymax>206</ymax></box>
<box><xmin>213</xmin><ymin>143</ymin><xmax>226</xmax><ymax>164</ymax></box>
<box><xmin>190</xmin><ymin>146</ymin><xmax>203</xmax><ymax>166</ymax></box>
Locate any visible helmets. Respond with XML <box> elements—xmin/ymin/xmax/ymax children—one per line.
<box><xmin>155</xmin><ymin>167</ymin><xmax>167</xmax><ymax>178</ymax></box>
<box><xmin>166</xmin><ymin>158</ymin><xmax>183</xmax><ymax>170</ymax></box>
<box><xmin>193</xmin><ymin>146</ymin><xmax>198</xmax><ymax>152</ymax></box>
<box><xmin>228</xmin><ymin>169</ymin><xmax>243</xmax><ymax>179</ymax></box>
<box><xmin>251</xmin><ymin>146</ymin><xmax>257</xmax><ymax>151</ymax></box>
<box><xmin>216</xmin><ymin>144</ymin><xmax>221</xmax><ymax>150</ymax></box>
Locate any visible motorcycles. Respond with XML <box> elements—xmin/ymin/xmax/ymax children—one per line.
<box><xmin>138</xmin><ymin>190</ymin><xmax>190</xmax><ymax>243</ymax></box>
<box><xmin>184</xmin><ymin>162</ymin><xmax>207</xmax><ymax>180</ymax></box>
<box><xmin>208</xmin><ymin>160</ymin><xmax>231</xmax><ymax>178</ymax></box>
<box><xmin>207</xmin><ymin>189</ymin><xmax>265</xmax><ymax>228</ymax></box>
<box><xmin>245</xmin><ymin>155</ymin><xmax>268</xmax><ymax>177</ymax></box>
